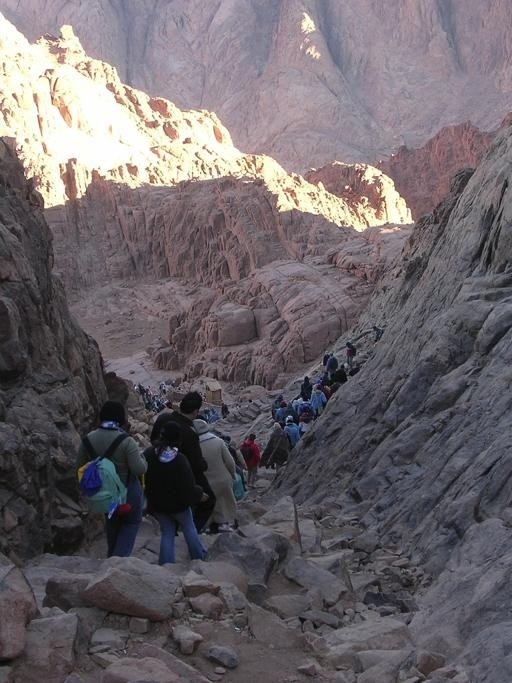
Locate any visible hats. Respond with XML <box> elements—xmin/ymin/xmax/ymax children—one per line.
<box><xmin>286</xmin><ymin>415</ymin><xmax>293</xmax><ymax>423</ymax></box>
<box><xmin>100</xmin><ymin>401</ymin><xmax>125</xmax><ymax>427</ymax></box>
<box><xmin>191</xmin><ymin>419</ymin><xmax>209</xmax><ymax>434</ymax></box>
<box><xmin>159</xmin><ymin>421</ymin><xmax>182</xmax><ymax>441</ymax></box>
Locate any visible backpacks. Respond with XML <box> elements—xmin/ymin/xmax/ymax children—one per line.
<box><xmin>77</xmin><ymin>433</ymin><xmax>133</xmax><ymax>520</ymax></box>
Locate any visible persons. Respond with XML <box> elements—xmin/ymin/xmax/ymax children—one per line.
<box><xmin>372</xmin><ymin>325</ymin><xmax>383</xmax><ymax>342</ymax></box>
<box><xmin>240</xmin><ymin>342</ymin><xmax>357</xmax><ymax>490</ymax></box>
<box><xmin>78</xmin><ymin>391</ymin><xmax>246</xmax><ymax>565</ymax></box>
<box><xmin>135</xmin><ymin>383</ymin><xmax>166</xmax><ymax>413</ymax></box>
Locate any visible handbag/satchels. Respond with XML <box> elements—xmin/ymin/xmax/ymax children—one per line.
<box><xmin>146</xmin><ymin>486</ymin><xmax>166</xmax><ymax>517</ymax></box>
